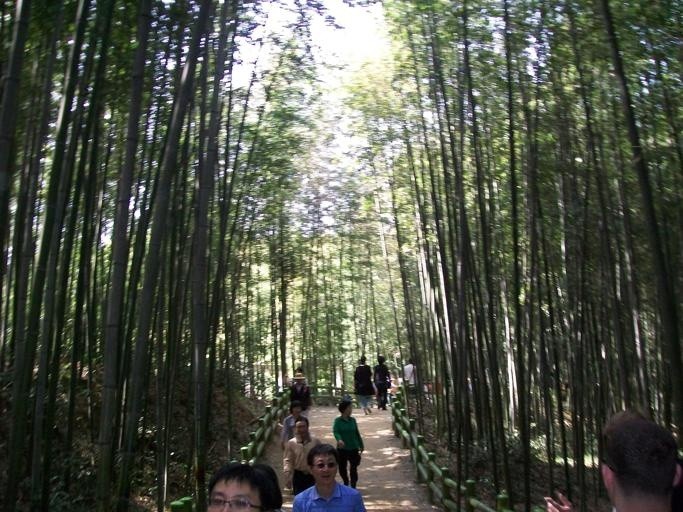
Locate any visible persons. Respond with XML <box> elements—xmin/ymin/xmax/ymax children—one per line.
<box><xmin>371</xmin><ymin>377</ymin><xmax>432</xmax><ymax>407</ymax></box>
<box><xmin>332</xmin><ymin>399</ymin><xmax>364</xmax><ymax>489</ymax></box>
<box><xmin>292</xmin><ymin>443</ymin><xmax>367</xmax><ymax>512</ymax></box>
<box><xmin>540</xmin><ymin>408</ymin><xmax>683</xmax><ymax>512</ymax></box>
<box><xmin>282</xmin><ymin>416</ymin><xmax>323</xmax><ymax>495</ymax></box>
<box><xmin>353</xmin><ymin>355</ymin><xmax>377</xmax><ymax>415</ymax></box>
<box><xmin>290</xmin><ymin>367</ymin><xmax>312</xmax><ymax>417</ymax></box>
<box><xmin>404</xmin><ymin>357</ymin><xmax>418</xmax><ymax>386</ymax></box>
<box><xmin>344</xmin><ymin>390</ymin><xmax>353</xmax><ymax>400</ymax></box>
<box><xmin>372</xmin><ymin>356</ymin><xmax>393</xmax><ymax>411</ymax></box>
<box><xmin>280</xmin><ymin>400</ymin><xmax>310</xmax><ymax>450</ymax></box>
<box><xmin>206</xmin><ymin>462</ymin><xmax>283</xmax><ymax>512</ymax></box>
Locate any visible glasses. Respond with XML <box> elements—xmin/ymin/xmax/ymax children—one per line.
<box><xmin>313</xmin><ymin>462</ymin><xmax>336</xmax><ymax>468</ymax></box>
<box><xmin>208</xmin><ymin>495</ymin><xmax>262</xmax><ymax>511</ymax></box>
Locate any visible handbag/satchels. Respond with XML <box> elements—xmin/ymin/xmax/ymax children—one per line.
<box><xmin>386</xmin><ymin>376</ymin><xmax>391</xmax><ymax>387</ymax></box>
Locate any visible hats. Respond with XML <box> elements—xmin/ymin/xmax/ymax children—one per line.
<box><xmin>293</xmin><ymin>373</ymin><xmax>306</xmax><ymax>380</ymax></box>
